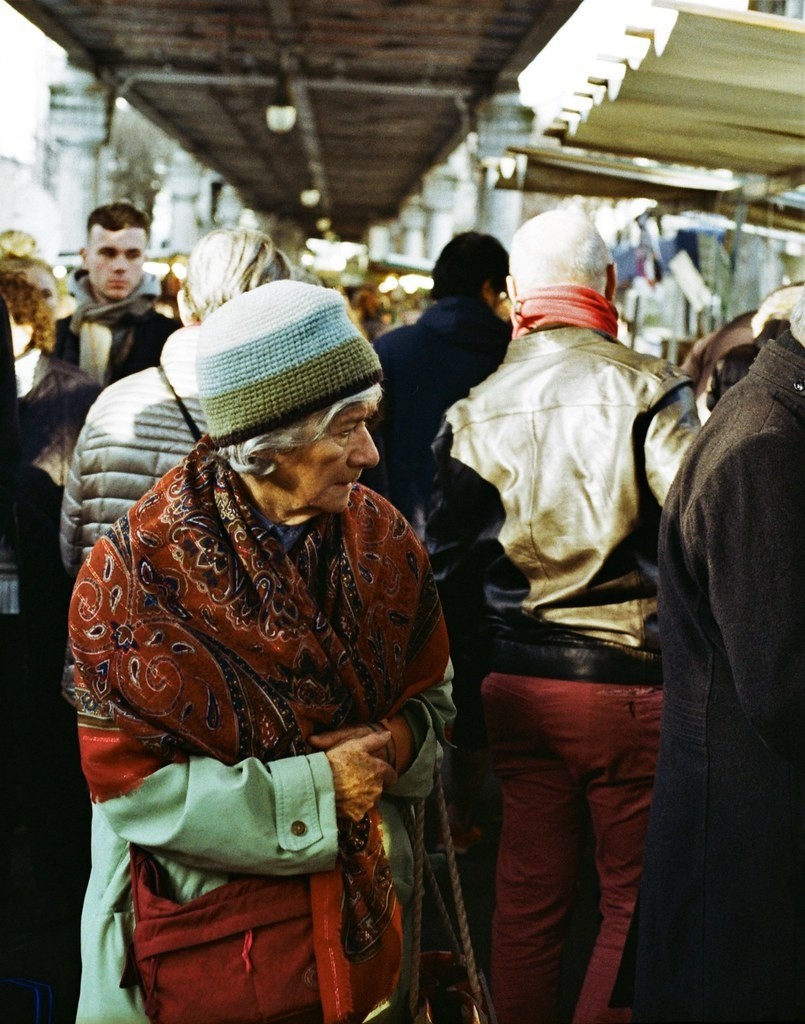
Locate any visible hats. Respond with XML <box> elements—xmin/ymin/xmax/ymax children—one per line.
<box><xmin>194</xmin><ymin>278</ymin><xmax>384</xmax><ymax>447</ymax></box>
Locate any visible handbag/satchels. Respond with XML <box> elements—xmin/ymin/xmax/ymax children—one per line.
<box><xmin>133</xmin><ymin>850</ymin><xmax>323</xmax><ymax>1024</ymax></box>
<box><xmin>410</xmin><ymin>952</ymin><xmax>482</xmax><ymax>1024</ymax></box>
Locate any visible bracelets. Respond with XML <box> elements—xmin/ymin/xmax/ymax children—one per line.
<box><xmin>364</xmin><ymin>725</ymin><xmax>389</xmax><ymax>769</ymax></box>
<box><xmin>375</xmin><ymin>718</ymin><xmax>396</xmax><ymax>769</ymax></box>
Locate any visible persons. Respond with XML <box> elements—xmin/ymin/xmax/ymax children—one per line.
<box><xmin>0</xmin><ymin>200</ymin><xmax>182</xmax><ymax>616</ymax></box>
<box><xmin>656</xmin><ymin>281</ymin><xmax>805</xmax><ymax>1024</ymax></box>
<box><xmin>358</xmin><ymin>207</ymin><xmax>701</xmax><ymax>1021</ymax></box>
<box><xmin>58</xmin><ymin>224</ymin><xmax>318</xmax><ymax>1022</ymax></box>
<box><xmin>70</xmin><ymin>278</ymin><xmax>495</xmax><ymax>1021</ymax></box>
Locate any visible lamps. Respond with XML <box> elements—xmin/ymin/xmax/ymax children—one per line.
<box><xmin>299</xmin><ymin>173</ymin><xmax>320</xmax><ymax>208</ymax></box>
<box><xmin>265</xmin><ymin>67</ymin><xmax>298</xmax><ymax>134</ymax></box>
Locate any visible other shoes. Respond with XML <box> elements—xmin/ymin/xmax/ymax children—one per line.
<box><xmin>438</xmin><ymin>812</ymin><xmax>472</xmax><ymax>854</ymax></box>
